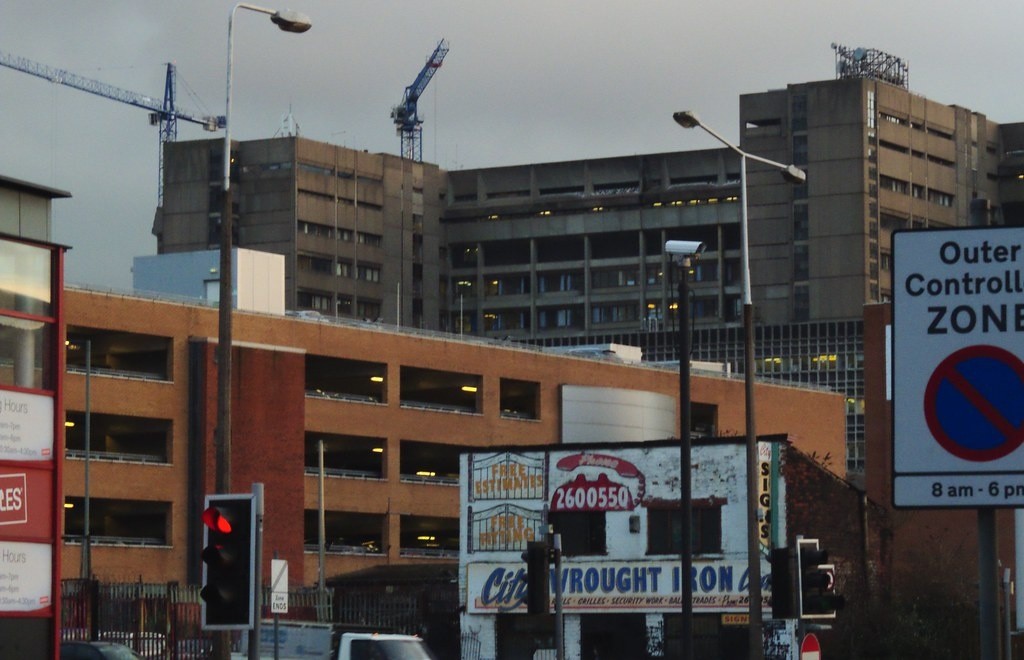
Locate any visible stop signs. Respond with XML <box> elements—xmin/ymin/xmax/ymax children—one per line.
<box><xmin>801</xmin><ymin>633</ymin><xmax>821</xmax><ymax>660</ymax></box>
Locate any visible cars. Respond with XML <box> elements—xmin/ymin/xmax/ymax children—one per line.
<box><xmin>60</xmin><ymin>641</ymin><xmax>146</xmax><ymax>660</ymax></box>
<box><xmin>98</xmin><ymin>631</ymin><xmax>165</xmax><ymax>655</ymax></box>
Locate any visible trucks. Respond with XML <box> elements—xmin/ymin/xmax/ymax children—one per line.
<box><xmin>241</xmin><ymin>621</ymin><xmax>436</xmax><ymax>660</ymax></box>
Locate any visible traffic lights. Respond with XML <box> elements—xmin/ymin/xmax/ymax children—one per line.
<box><xmin>796</xmin><ymin>537</ymin><xmax>837</xmax><ymax>619</ymax></box>
<box><xmin>199</xmin><ymin>494</ymin><xmax>256</xmax><ymax>630</ymax></box>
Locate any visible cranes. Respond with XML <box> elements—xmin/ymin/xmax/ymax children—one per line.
<box><xmin>0</xmin><ymin>51</ymin><xmax>226</xmax><ymax>208</ymax></box>
<box><xmin>390</xmin><ymin>37</ymin><xmax>451</xmax><ymax>161</ymax></box>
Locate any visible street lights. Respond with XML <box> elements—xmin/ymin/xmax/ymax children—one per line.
<box><xmin>672</xmin><ymin>110</ymin><xmax>805</xmax><ymax>660</ymax></box>
<box><xmin>214</xmin><ymin>3</ymin><xmax>311</xmax><ymax>493</ymax></box>
